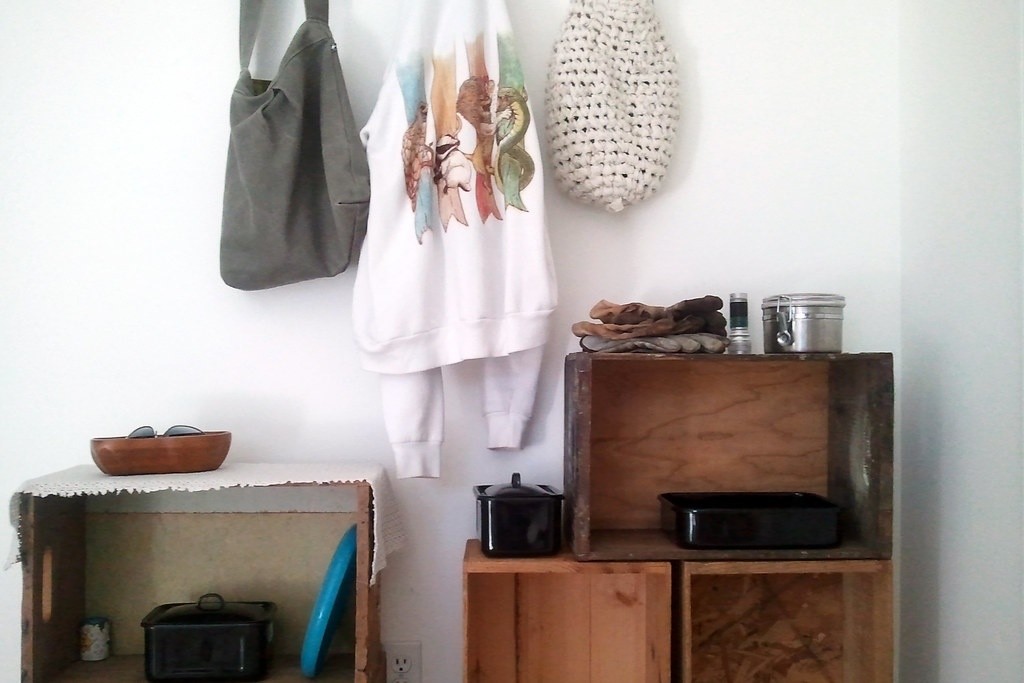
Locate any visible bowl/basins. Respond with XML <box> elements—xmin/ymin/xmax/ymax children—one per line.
<box><xmin>761</xmin><ymin>292</ymin><xmax>846</xmax><ymax>353</ymax></box>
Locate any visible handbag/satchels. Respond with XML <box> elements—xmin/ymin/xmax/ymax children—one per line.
<box><xmin>220</xmin><ymin>1</ymin><xmax>368</xmax><ymax>290</ymax></box>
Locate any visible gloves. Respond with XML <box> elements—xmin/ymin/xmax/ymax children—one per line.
<box><xmin>572</xmin><ymin>296</ymin><xmax>729</xmax><ymax>353</ymax></box>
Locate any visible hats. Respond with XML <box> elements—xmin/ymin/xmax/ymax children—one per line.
<box><xmin>546</xmin><ymin>0</ymin><xmax>681</xmax><ymax>210</ymax></box>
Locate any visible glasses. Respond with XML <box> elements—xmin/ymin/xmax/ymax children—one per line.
<box><xmin>126</xmin><ymin>425</ymin><xmax>206</xmax><ymax>438</ymax></box>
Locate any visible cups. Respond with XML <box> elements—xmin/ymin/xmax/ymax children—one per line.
<box><xmin>81</xmin><ymin>617</ymin><xmax>111</xmax><ymax>661</ymax></box>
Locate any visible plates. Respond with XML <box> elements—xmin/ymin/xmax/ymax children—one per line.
<box><xmin>298</xmin><ymin>523</ymin><xmax>356</xmax><ymax>677</ymax></box>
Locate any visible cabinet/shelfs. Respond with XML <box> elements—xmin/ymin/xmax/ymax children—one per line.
<box><xmin>681</xmin><ymin>557</ymin><xmax>897</xmax><ymax>683</ymax></box>
<box><xmin>562</xmin><ymin>351</ymin><xmax>895</xmax><ymax>561</ymax></box>
<box><xmin>463</xmin><ymin>540</ymin><xmax>674</xmax><ymax>683</ymax></box>
<box><xmin>10</xmin><ymin>465</ymin><xmax>406</xmax><ymax>683</ymax></box>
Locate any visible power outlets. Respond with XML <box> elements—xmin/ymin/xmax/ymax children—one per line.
<box><xmin>384</xmin><ymin>642</ymin><xmax>423</xmax><ymax>682</ymax></box>
